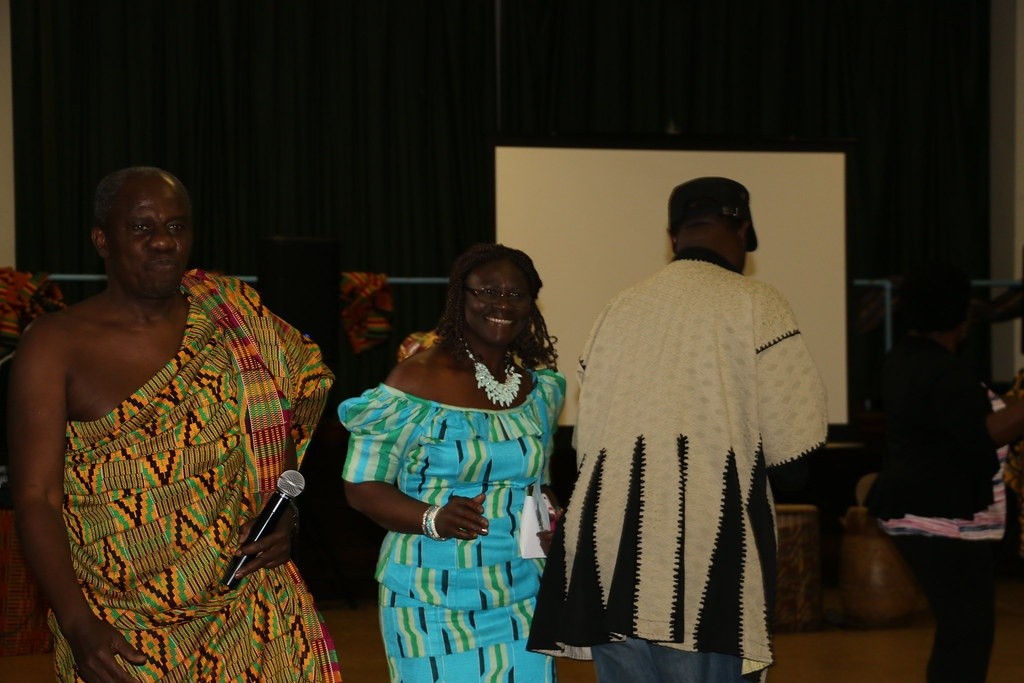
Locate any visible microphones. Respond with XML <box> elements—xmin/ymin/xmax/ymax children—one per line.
<box><xmin>220</xmin><ymin>469</ymin><xmax>306</xmax><ymax>590</ymax></box>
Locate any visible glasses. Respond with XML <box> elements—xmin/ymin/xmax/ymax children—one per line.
<box><xmin>462</xmin><ymin>284</ymin><xmax>532</xmax><ymax>301</ymax></box>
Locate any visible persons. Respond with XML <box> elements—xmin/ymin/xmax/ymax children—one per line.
<box><xmin>527</xmin><ymin>175</ymin><xmax>833</xmax><ymax>682</ymax></box>
<box><xmin>335</xmin><ymin>243</ymin><xmax>568</xmax><ymax>683</ymax></box>
<box><xmin>874</xmin><ymin>258</ymin><xmax>1023</xmax><ymax>682</ymax></box>
<box><xmin>5</xmin><ymin>162</ymin><xmax>337</xmax><ymax>682</ymax></box>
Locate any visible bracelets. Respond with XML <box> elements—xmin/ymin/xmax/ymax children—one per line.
<box><xmin>431</xmin><ymin>507</ymin><xmax>449</xmax><ymax>541</ymax></box>
<box><xmin>422</xmin><ymin>505</ymin><xmax>436</xmax><ymax>537</ymax></box>
<box><xmin>426</xmin><ymin>506</ymin><xmax>441</xmax><ymax>541</ymax></box>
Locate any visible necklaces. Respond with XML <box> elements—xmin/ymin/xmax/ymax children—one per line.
<box><xmin>459</xmin><ymin>334</ymin><xmax>521</xmax><ymax>407</ymax></box>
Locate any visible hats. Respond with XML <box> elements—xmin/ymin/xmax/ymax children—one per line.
<box><xmin>668</xmin><ymin>176</ymin><xmax>757</xmax><ymax>252</ymax></box>
